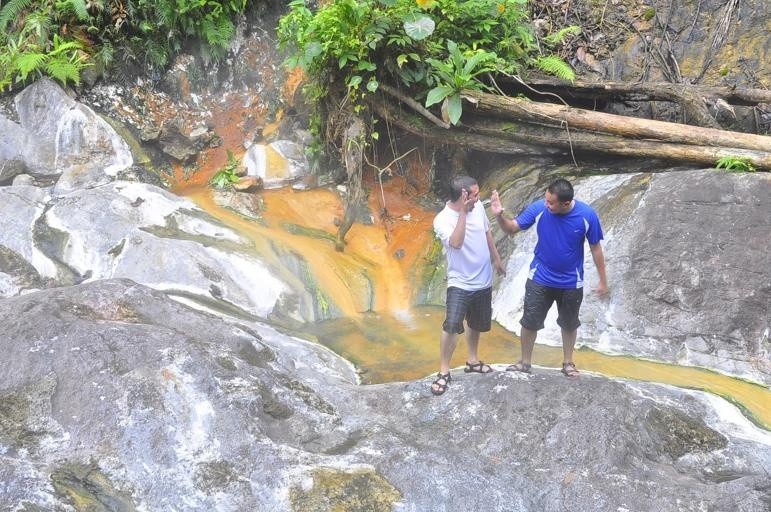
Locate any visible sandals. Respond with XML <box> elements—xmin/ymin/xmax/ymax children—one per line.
<box><xmin>506</xmin><ymin>359</ymin><xmax>532</xmax><ymax>374</ymax></box>
<box><xmin>430</xmin><ymin>371</ymin><xmax>453</xmax><ymax>396</ymax></box>
<box><xmin>561</xmin><ymin>361</ymin><xmax>580</xmax><ymax>380</ymax></box>
<box><xmin>464</xmin><ymin>361</ymin><xmax>494</xmax><ymax>373</ymax></box>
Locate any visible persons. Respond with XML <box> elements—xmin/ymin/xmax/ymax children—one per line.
<box><xmin>489</xmin><ymin>179</ymin><xmax>612</xmax><ymax>379</ymax></box>
<box><xmin>430</xmin><ymin>174</ymin><xmax>509</xmax><ymax>396</ymax></box>
